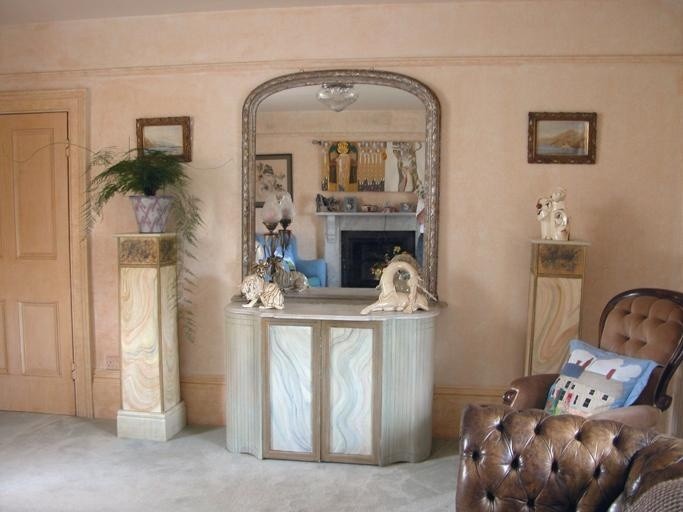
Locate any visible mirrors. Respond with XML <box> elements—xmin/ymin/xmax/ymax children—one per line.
<box><xmin>240</xmin><ymin>70</ymin><xmax>441</xmax><ymax>301</ymax></box>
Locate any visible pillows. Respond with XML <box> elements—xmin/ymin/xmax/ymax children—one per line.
<box><xmin>541</xmin><ymin>338</ymin><xmax>669</xmax><ymax>416</ymax></box>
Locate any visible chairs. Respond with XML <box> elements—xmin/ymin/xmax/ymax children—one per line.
<box><xmin>253</xmin><ymin>232</ymin><xmax>327</xmax><ymax>287</ymax></box>
<box><xmin>502</xmin><ymin>286</ymin><xmax>682</xmax><ymax>427</ymax></box>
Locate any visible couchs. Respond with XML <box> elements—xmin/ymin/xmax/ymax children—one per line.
<box><xmin>450</xmin><ymin>401</ymin><xmax>683</xmax><ymax>512</ymax></box>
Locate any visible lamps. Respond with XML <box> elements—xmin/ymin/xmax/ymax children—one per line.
<box><xmin>314</xmin><ymin>76</ymin><xmax>359</xmax><ymax>114</ymax></box>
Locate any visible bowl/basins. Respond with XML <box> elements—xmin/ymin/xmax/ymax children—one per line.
<box><xmin>360</xmin><ymin>204</ymin><xmax>377</xmax><ymax>212</ymax></box>
<box><xmin>384</xmin><ymin>207</ymin><xmax>396</xmax><ymax>213</ymax></box>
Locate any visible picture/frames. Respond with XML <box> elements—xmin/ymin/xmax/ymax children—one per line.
<box><xmin>255</xmin><ymin>154</ymin><xmax>293</xmax><ymax>209</ymax></box>
<box><xmin>136</xmin><ymin>117</ymin><xmax>191</xmax><ymax>163</ymax></box>
<box><xmin>527</xmin><ymin>112</ymin><xmax>596</xmax><ymax>164</ymax></box>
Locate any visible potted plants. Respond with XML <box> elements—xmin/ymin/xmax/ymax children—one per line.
<box><xmin>74</xmin><ymin>136</ymin><xmax>207</xmax><ymax>347</ymax></box>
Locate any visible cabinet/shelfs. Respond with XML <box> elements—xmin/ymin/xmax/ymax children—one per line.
<box><xmin>225</xmin><ymin>291</ymin><xmax>442</xmax><ymax>468</ymax></box>
